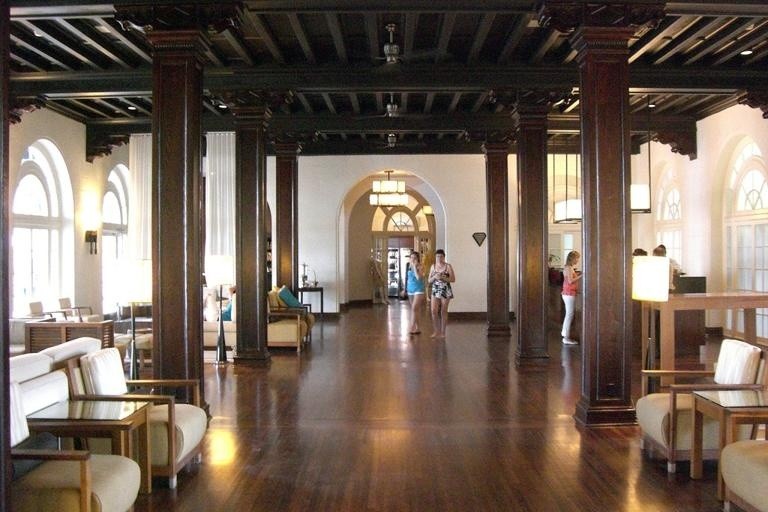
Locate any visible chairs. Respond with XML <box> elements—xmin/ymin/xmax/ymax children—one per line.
<box><xmin>7</xmin><ymin>297</ymin><xmax>154</xmax><ymax>427</ymax></box>
<box><xmin>203</xmin><ymin>285</ymin><xmax>238</xmax><ymax>349</ymax></box>
<box><xmin>635</xmin><ymin>337</ymin><xmax>764</xmax><ymax>472</ymax></box>
<box><xmin>67</xmin><ymin>347</ymin><xmax>206</xmax><ymax>489</ymax></box>
<box><xmin>267</xmin><ymin>284</ymin><xmax>314</xmax><ymax>353</ymax></box>
<box><xmin>720</xmin><ymin>414</ymin><xmax>768</xmax><ymax>510</ymax></box>
<box><xmin>7</xmin><ymin>382</ymin><xmax>141</xmax><ymax>512</ymax></box>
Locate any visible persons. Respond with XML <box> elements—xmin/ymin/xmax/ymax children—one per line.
<box><xmin>371</xmin><ymin>259</ymin><xmax>392</xmax><ymax>305</ymax></box>
<box><xmin>653</xmin><ymin>244</ymin><xmax>680</xmax><ymax>293</ymax></box>
<box><xmin>427</xmin><ymin>249</ymin><xmax>455</xmax><ymax>341</ymax></box>
<box><xmin>560</xmin><ymin>250</ymin><xmax>583</xmax><ymax>346</ymax></box>
<box><xmin>407</xmin><ymin>252</ymin><xmax>425</xmax><ymax>334</ymax></box>
<box><xmin>204</xmin><ymin>286</ymin><xmax>236</xmax><ymax>323</ymax></box>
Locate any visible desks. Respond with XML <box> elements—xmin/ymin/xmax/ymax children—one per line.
<box><xmin>27</xmin><ymin>400</ymin><xmax>151</xmax><ymax>499</ymax></box>
<box><xmin>690</xmin><ymin>389</ymin><xmax>768</xmax><ymax>499</ymax></box>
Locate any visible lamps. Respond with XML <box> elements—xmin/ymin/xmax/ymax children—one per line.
<box><xmin>386</xmin><ymin>92</ymin><xmax>399</xmax><ymax>118</ymax></box>
<box><xmin>630</xmin><ymin>94</ymin><xmax>651</xmax><ymax>213</ymax></box>
<box><xmin>384</xmin><ymin>23</ymin><xmax>400</xmax><ymax>65</ymax></box>
<box><xmin>554</xmin><ymin>127</ymin><xmax>584</xmax><ymax>225</ymax></box>
<box><xmin>369</xmin><ymin>170</ymin><xmax>409</xmax><ymax>207</ymax></box>
<box><xmin>386</xmin><ymin>126</ymin><xmax>398</xmax><ymax>149</ymax></box>
<box><xmin>84</xmin><ymin>230</ymin><xmax>99</xmax><ymax>255</ymax></box>
<box><xmin>630</xmin><ymin>254</ymin><xmax>668</xmax><ymax>303</ymax></box>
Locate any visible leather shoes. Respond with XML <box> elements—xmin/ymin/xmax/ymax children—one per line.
<box><xmin>562</xmin><ymin>338</ymin><xmax>578</xmax><ymax>344</ymax></box>
